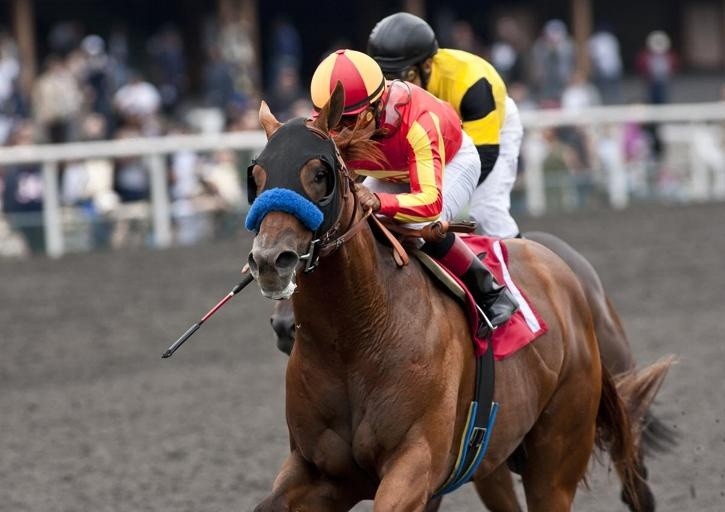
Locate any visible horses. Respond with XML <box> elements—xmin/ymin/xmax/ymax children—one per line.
<box><xmin>239</xmin><ymin>79</ymin><xmax>679</xmax><ymax>512</ymax></box>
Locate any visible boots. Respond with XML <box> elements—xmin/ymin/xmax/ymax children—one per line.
<box><xmin>421</xmin><ymin>228</ymin><xmax>520</xmax><ymax>339</ymax></box>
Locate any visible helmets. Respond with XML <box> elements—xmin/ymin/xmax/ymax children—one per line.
<box><xmin>312</xmin><ymin>48</ymin><xmax>386</xmax><ymax>116</ymax></box>
<box><xmin>367</xmin><ymin>12</ymin><xmax>438</xmax><ymax>72</ymax></box>
